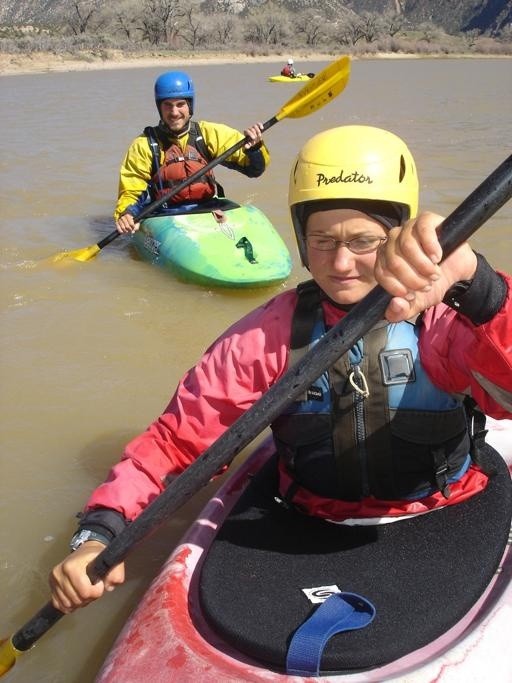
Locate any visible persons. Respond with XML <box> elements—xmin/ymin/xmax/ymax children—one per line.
<box><xmin>280</xmin><ymin>58</ymin><xmax>294</xmax><ymax>76</ymax></box>
<box><xmin>49</xmin><ymin>125</ymin><xmax>512</xmax><ymax>617</ymax></box>
<box><xmin>113</xmin><ymin>69</ymin><xmax>271</xmax><ymax>235</ymax></box>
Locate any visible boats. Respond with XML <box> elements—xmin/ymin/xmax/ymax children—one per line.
<box><xmin>84</xmin><ymin>418</ymin><xmax>512</xmax><ymax>681</ymax></box>
<box><xmin>118</xmin><ymin>192</ymin><xmax>297</xmax><ymax>291</ymax></box>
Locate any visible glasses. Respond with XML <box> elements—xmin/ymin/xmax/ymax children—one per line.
<box><xmin>298</xmin><ymin>230</ymin><xmax>387</xmax><ymax>254</ymax></box>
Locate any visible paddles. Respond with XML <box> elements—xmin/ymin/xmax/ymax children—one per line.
<box><xmin>36</xmin><ymin>55</ymin><xmax>351</xmax><ymax>270</ymax></box>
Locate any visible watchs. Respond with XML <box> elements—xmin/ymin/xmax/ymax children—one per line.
<box><xmin>69</xmin><ymin>528</ymin><xmax>110</xmax><ymax>552</ymax></box>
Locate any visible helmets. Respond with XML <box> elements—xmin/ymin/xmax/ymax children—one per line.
<box><xmin>287</xmin><ymin>124</ymin><xmax>421</xmax><ymax>268</ymax></box>
<box><xmin>153</xmin><ymin>72</ymin><xmax>197</xmax><ymax>115</ymax></box>
